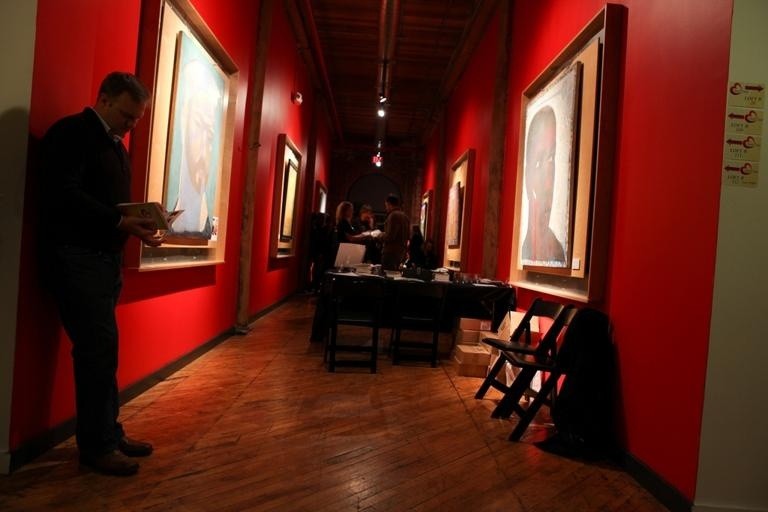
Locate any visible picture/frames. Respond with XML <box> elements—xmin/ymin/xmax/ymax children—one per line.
<box><xmin>447</xmin><ymin>182</ymin><xmax>461</xmax><ymax>246</ymax></box>
<box><xmin>280</xmin><ymin>159</ymin><xmax>299</xmax><ymax>240</ymax></box>
<box><xmin>419</xmin><ymin>203</ymin><xmax>427</xmax><ymax>239</ymax></box>
<box><xmin>519</xmin><ymin>60</ymin><xmax>582</xmax><ymax>268</ymax></box>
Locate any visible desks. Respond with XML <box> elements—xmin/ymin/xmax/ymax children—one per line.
<box><xmin>310</xmin><ymin>268</ymin><xmax>515</xmax><ymax>362</ymax></box>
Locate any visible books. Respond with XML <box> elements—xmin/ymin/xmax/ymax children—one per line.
<box><xmin>118</xmin><ymin>202</ymin><xmax>186</xmax><ymax>230</ymax></box>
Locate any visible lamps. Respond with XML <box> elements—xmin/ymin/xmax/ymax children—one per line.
<box><xmin>378</xmin><ymin>59</ymin><xmax>388</xmax><ymax>103</ymax></box>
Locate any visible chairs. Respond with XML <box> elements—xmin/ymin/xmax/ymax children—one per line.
<box><xmin>474</xmin><ymin>298</ymin><xmax>575</xmax><ymax>418</ymax></box>
<box><xmin>389</xmin><ymin>281</ymin><xmax>449</xmax><ymax>368</ymax></box>
<box><xmin>324</xmin><ymin>275</ymin><xmax>385</xmax><ymax>373</ymax></box>
<box><xmin>502</xmin><ymin>303</ymin><xmax>578</xmax><ymax>442</ymax></box>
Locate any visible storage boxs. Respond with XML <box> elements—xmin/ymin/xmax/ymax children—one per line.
<box><xmin>452</xmin><ymin>310</ymin><xmax>542</xmax><ymax>402</ymax></box>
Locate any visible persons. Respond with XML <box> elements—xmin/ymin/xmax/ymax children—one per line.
<box><xmin>521</xmin><ymin>105</ymin><xmax>566</xmax><ymax>267</ymax></box>
<box><xmin>28</xmin><ymin>70</ymin><xmax>168</xmax><ymax>479</ymax></box>
<box><xmin>302</xmin><ymin>192</ymin><xmax>439</xmax><ymax>292</ymax></box>
<box><xmin>170</xmin><ymin>58</ymin><xmax>218</xmax><ymax>240</ymax></box>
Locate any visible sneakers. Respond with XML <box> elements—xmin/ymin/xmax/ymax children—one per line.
<box><xmin>79</xmin><ymin>447</ymin><xmax>140</xmax><ymax>476</ymax></box>
<box><xmin>112</xmin><ymin>433</ymin><xmax>153</xmax><ymax>457</ymax></box>
